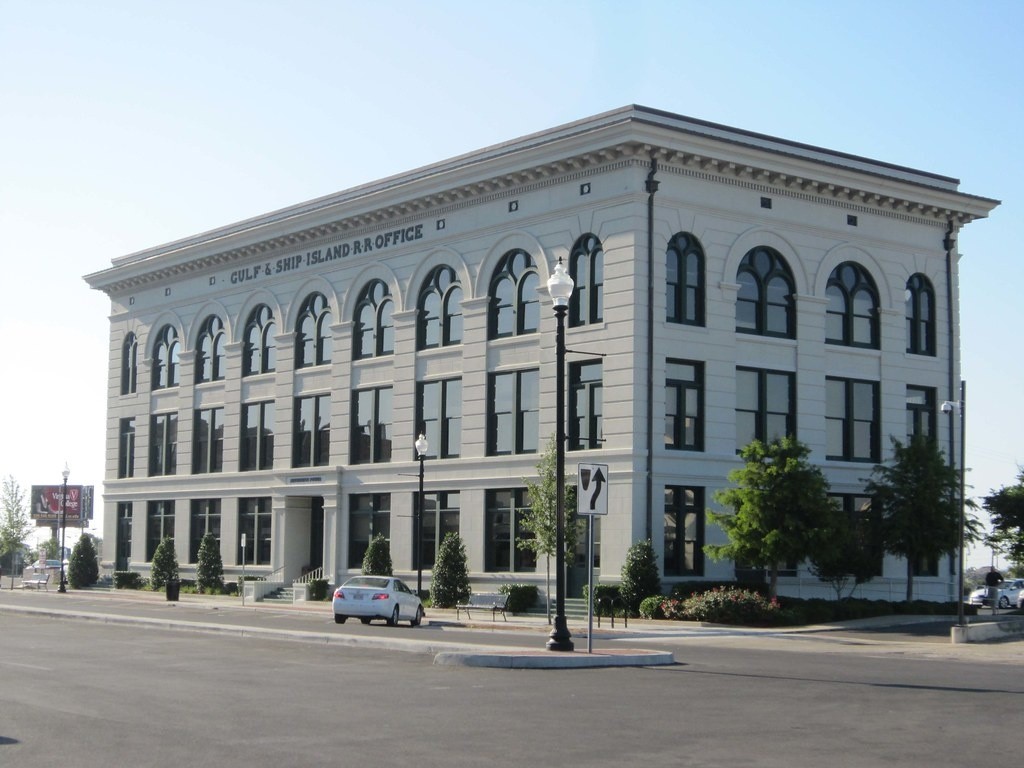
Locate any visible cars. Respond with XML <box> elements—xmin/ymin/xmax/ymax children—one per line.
<box><xmin>970</xmin><ymin>578</ymin><xmax>1024</xmax><ymax>609</ymax></box>
<box><xmin>332</xmin><ymin>576</ymin><xmax>424</xmax><ymax>627</ymax></box>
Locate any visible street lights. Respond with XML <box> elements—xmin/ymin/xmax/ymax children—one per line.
<box><xmin>58</xmin><ymin>461</ymin><xmax>70</xmax><ymax>593</ymax></box>
<box><xmin>546</xmin><ymin>256</ymin><xmax>575</xmax><ymax>652</ymax></box>
<box><xmin>415</xmin><ymin>430</ymin><xmax>428</xmax><ymax>598</ymax></box>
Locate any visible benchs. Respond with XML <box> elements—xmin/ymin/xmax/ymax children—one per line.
<box><xmin>22</xmin><ymin>573</ymin><xmax>51</xmax><ymax>593</ymax></box>
<box><xmin>455</xmin><ymin>592</ymin><xmax>511</xmax><ymax>622</ymax></box>
